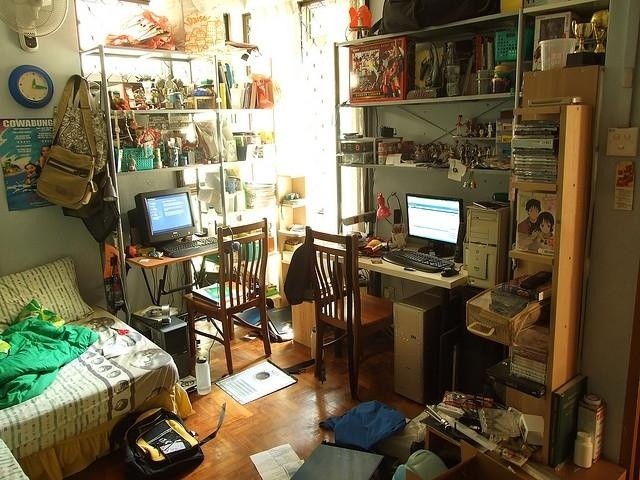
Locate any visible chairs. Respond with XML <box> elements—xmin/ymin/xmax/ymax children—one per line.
<box><xmin>182</xmin><ymin>218</ymin><xmax>272</xmax><ymax>375</ymax></box>
<box><xmin>306</xmin><ymin>226</ymin><xmax>393</xmax><ymax>400</ymax></box>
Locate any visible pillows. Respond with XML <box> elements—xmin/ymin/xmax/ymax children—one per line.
<box><xmin>0</xmin><ymin>256</ymin><xmax>93</xmax><ymax>334</ymax></box>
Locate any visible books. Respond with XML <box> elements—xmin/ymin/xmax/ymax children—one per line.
<box><xmin>511</xmin><ymin>119</ymin><xmax>559</xmax><ymax>185</ymax></box>
<box><xmin>510</xmin><ymin>325</ymin><xmax>547</xmax><ymax>384</ymax></box>
<box><xmin>503</xmin><ymin>274</ymin><xmax>552</xmax><ymax>301</ymax></box>
<box><xmin>243</xmin><ymin>179</ymin><xmax>276</xmax><ymax>209</ymax></box>
<box><xmin>425</xmin><ymin>391</ymin><xmax>494</xmax><ymax>426</ymax></box>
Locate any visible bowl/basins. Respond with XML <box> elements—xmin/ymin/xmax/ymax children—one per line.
<box><xmin>539</xmin><ymin>37</ymin><xmax>578</xmax><ymax>72</ymax></box>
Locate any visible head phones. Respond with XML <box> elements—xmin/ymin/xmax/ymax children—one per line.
<box><xmin>224</xmin><ymin>225</ymin><xmax>240</xmax><ymax>254</ymax></box>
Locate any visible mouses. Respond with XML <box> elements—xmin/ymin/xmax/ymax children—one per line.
<box><xmin>441</xmin><ymin>267</ymin><xmax>458</xmax><ymax>276</ymax></box>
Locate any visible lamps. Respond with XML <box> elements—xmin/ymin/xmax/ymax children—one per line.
<box><xmin>376</xmin><ymin>193</ymin><xmax>403</xmax><ymax>225</ymax></box>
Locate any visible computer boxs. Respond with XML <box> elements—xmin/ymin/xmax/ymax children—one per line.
<box><xmin>463</xmin><ymin>205</ymin><xmax>509</xmax><ymax>289</ymax></box>
<box><xmin>131</xmin><ymin>314</ymin><xmax>192</xmax><ymax>381</ymax></box>
<box><xmin>392</xmin><ymin>287</ymin><xmax>461</xmax><ymax>404</ymax></box>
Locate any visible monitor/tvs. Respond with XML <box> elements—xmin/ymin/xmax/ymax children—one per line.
<box><xmin>127</xmin><ymin>187</ymin><xmax>196</xmax><ymax>247</ymax></box>
<box><xmin>405</xmin><ymin>193</ymin><xmax>462</xmax><ymax>255</ymax></box>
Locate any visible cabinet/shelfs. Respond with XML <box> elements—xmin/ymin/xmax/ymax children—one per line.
<box><xmin>80</xmin><ymin>44</ymin><xmax>280</xmax><ymax>326</ymax></box>
<box><xmin>506</xmin><ymin>104</ymin><xmax>594</xmax><ymax>468</ymax></box>
<box><xmin>334</xmin><ymin>0</ymin><xmax>609</xmax><ymax>294</ymax></box>
<box><xmin>275</xmin><ymin>175</ymin><xmax>307</xmax><ymax>309</ymax></box>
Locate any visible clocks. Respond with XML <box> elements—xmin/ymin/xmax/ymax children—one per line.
<box><xmin>8</xmin><ymin>65</ymin><xmax>54</xmax><ymax>109</ymax></box>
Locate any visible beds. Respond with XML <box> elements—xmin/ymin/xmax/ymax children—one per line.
<box><xmin>0</xmin><ymin>303</ymin><xmax>181</xmax><ymax>480</ymax></box>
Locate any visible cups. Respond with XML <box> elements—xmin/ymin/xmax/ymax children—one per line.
<box><xmin>169</xmin><ymin>92</ymin><xmax>184</xmax><ymax>111</ymax></box>
<box><xmin>380</xmin><ymin>126</ymin><xmax>396</xmax><ymax>137</ymax></box>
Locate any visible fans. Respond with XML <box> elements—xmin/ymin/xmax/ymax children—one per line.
<box><xmin>0</xmin><ymin>0</ymin><xmax>68</xmax><ymax>53</ymax></box>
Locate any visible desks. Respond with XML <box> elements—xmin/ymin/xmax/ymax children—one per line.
<box><xmin>420</xmin><ymin>417</ymin><xmax>627</xmax><ymax>480</ymax></box>
<box><xmin>333</xmin><ymin>245</ymin><xmax>468</xmax><ymax>357</ymax></box>
<box><xmin>126</xmin><ymin>236</ymin><xmax>236</xmax><ymax>326</ymax></box>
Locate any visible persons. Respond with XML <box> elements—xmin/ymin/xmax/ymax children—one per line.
<box><xmin>527</xmin><ymin>212</ymin><xmax>556</xmax><ymax>255</ymax></box>
<box><xmin>518</xmin><ymin>198</ymin><xmax>542</xmax><ymax>250</ymax></box>
<box><xmin>39</xmin><ymin>145</ymin><xmax>52</xmax><ymax>169</ymax></box>
<box><xmin>24</xmin><ymin>161</ymin><xmax>41</xmax><ymax>189</ymax></box>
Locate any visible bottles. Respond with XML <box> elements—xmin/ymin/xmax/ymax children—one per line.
<box><xmin>445</xmin><ymin>67</ymin><xmax>460</xmax><ymax>98</ymax></box>
<box><xmin>573</xmin><ymin>431</ymin><xmax>594</xmax><ymax>469</ymax></box>
<box><xmin>231</xmin><ymin>132</ymin><xmax>248</xmax><ymax>161</ymax></box>
<box><xmin>194</xmin><ymin>355</ymin><xmax>212</xmax><ymax>396</ymax></box>
<box><xmin>153</xmin><ymin>146</ymin><xmax>163</xmax><ymax>169</ymax></box>
<box><xmin>490</xmin><ymin>65</ymin><xmax>512</xmax><ymax>94</ymax></box>
<box><xmin>168</xmin><ymin>145</ymin><xmax>180</xmax><ymax>168</ymax></box>
<box><xmin>578</xmin><ymin>393</ymin><xmax>606</xmax><ymax>463</ymax></box>
<box><xmin>476</xmin><ymin>69</ymin><xmax>491</xmax><ymax>95</ymax></box>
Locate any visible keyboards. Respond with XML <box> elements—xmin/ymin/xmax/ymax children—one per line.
<box><xmin>161</xmin><ymin>236</ymin><xmax>224</xmax><ymax>257</ymax></box>
<box><xmin>382</xmin><ymin>250</ymin><xmax>453</xmax><ymax>273</ymax></box>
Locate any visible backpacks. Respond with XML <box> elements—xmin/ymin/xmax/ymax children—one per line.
<box><xmin>114</xmin><ymin>403</ymin><xmax>226</xmax><ymax>480</ymax></box>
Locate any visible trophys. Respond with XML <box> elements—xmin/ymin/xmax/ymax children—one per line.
<box><xmin>591</xmin><ymin>9</ymin><xmax>610</xmax><ymax>53</ymax></box>
<box><xmin>571</xmin><ymin>20</ymin><xmax>594</xmax><ymax>53</ymax></box>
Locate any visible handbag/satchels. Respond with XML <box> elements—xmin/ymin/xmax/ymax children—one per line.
<box><xmin>35</xmin><ymin>75</ymin><xmax>120</xmax><ymax>244</ymax></box>
